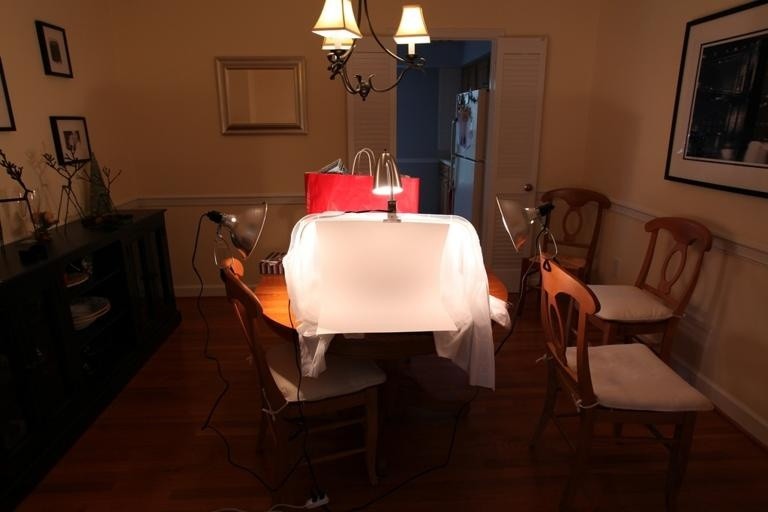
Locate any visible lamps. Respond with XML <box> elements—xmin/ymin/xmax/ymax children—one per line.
<box><xmin>371</xmin><ymin>149</ymin><xmax>404</xmax><ymax>222</ymax></box>
<box><xmin>203</xmin><ymin>201</ymin><xmax>269</xmax><ymax>276</ymax></box>
<box><xmin>493</xmin><ymin>184</ymin><xmax>563</xmax><ymax>272</ymax></box>
<box><xmin>312</xmin><ymin>0</ymin><xmax>431</xmax><ymax>102</ymax></box>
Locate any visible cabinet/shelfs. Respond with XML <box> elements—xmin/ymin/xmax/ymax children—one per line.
<box><xmin>0</xmin><ymin>209</ymin><xmax>184</xmax><ymax>511</ymax></box>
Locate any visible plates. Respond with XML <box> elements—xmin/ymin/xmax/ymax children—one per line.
<box><xmin>73</xmin><ymin>296</ymin><xmax>111</xmax><ymax>325</ymax></box>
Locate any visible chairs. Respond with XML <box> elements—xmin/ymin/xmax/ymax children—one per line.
<box><xmin>564</xmin><ymin>217</ymin><xmax>712</xmax><ymax>363</ymax></box>
<box><xmin>220</xmin><ymin>268</ymin><xmax>386</xmax><ymax>483</ymax></box>
<box><xmin>516</xmin><ymin>186</ymin><xmax>614</xmax><ymax>321</ymax></box>
<box><xmin>528</xmin><ymin>252</ymin><xmax>715</xmax><ymax>512</ymax></box>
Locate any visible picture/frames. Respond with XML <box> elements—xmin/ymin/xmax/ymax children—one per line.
<box><xmin>0</xmin><ymin>58</ymin><xmax>18</xmax><ymax>132</ymax></box>
<box><xmin>663</xmin><ymin>0</ymin><xmax>768</xmax><ymax>202</ymax></box>
<box><xmin>0</xmin><ymin>197</ymin><xmax>36</xmax><ymax>248</ymax></box>
<box><xmin>34</xmin><ymin>20</ymin><xmax>74</xmax><ymax>79</ymax></box>
<box><xmin>48</xmin><ymin>115</ymin><xmax>93</xmax><ymax>166</ymax></box>
<box><xmin>215</xmin><ymin>55</ymin><xmax>308</xmax><ymax>138</ymax></box>
